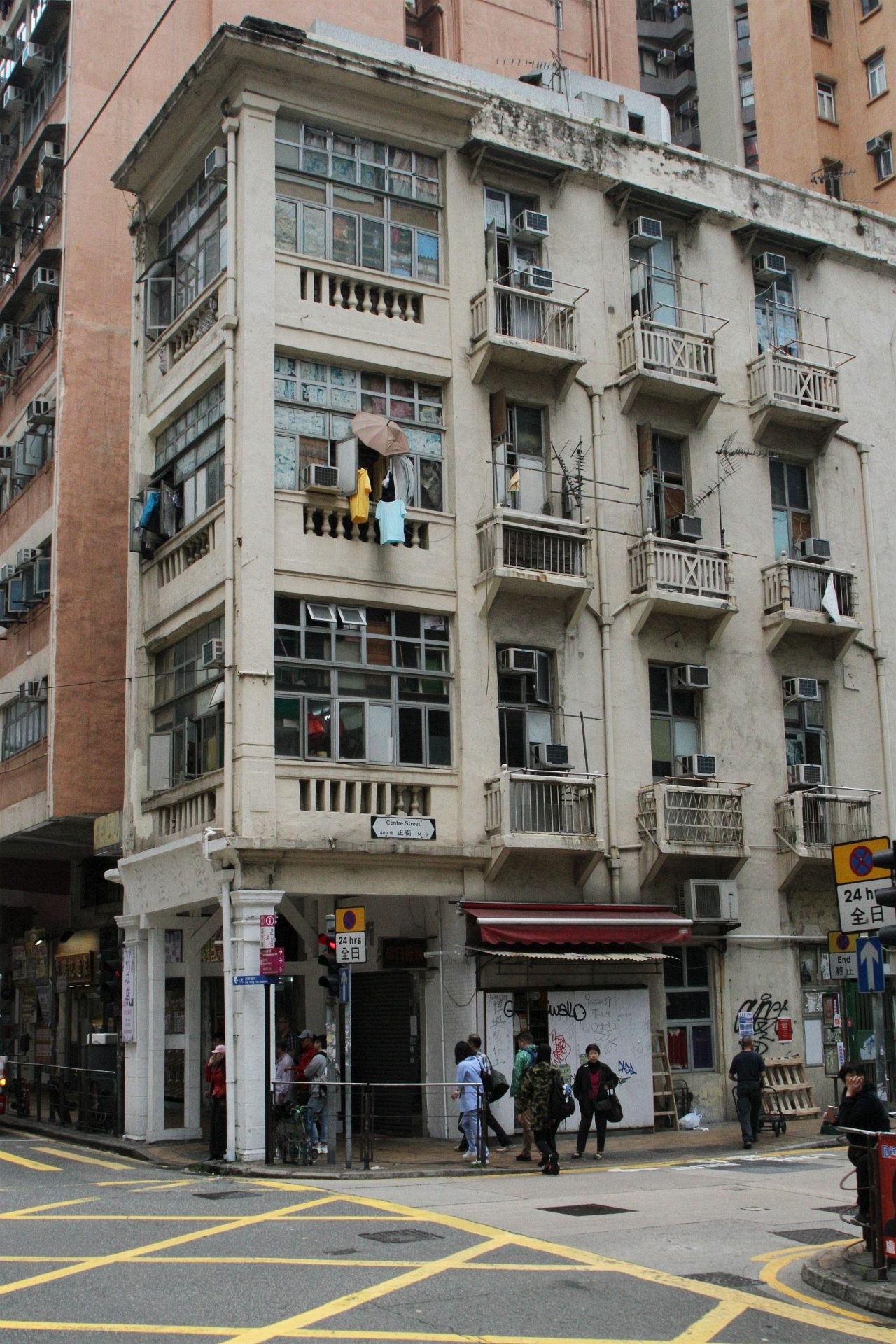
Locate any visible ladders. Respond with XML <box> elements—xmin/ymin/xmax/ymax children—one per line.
<box><xmin>653</xmin><ymin>1030</ymin><xmax>679</xmax><ymax>1129</ymax></box>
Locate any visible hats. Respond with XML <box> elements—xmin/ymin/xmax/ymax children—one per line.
<box><xmin>298</xmin><ymin>1029</ymin><xmax>313</xmax><ymax>1039</ymax></box>
<box><xmin>212</xmin><ymin>1044</ymin><xmax>226</xmax><ymax>1055</ymax></box>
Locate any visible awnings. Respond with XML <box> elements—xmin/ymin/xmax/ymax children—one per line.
<box><xmin>462</xmin><ymin>906</ymin><xmax>693</xmax><ymax>960</ymax></box>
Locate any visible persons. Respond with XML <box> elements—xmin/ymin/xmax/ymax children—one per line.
<box><xmin>451</xmin><ymin>1034</ymin><xmax>510</xmax><ymax>1166</ymax></box>
<box><xmin>209</xmin><ymin>1026</ymin><xmax>327</xmax><ymax>1170</ymax></box>
<box><xmin>514</xmin><ymin>1044</ymin><xmax>566</xmax><ymax>1177</ymax></box>
<box><xmin>728</xmin><ymin>1034</ymin><xmax>765</xmax><ymax>1150</ymax></box>
<box><xmin>510</xmin><ymin>1029</ymin><xmax>538</xmax><ymax>1164</ymax></box>
<box><xmin>572</xmin><ymin>1045</ymin><xmax>623</xmax><ymax>1159</ymax></box>
<box><xmin>46</xmin><ymin>1078</ymin><xmax>78</xmax><ymax>1124</ymax></box>
<box><xmin>823</xmin><ymin>1061</ymin><xmax>891</xmax><ymax>1232</ymax></box>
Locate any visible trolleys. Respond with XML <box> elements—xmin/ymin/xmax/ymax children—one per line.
<box><xmin>731</xmin><ymin>1085</ymin><xmax>787</xmax><ymax>1138</ymax></box>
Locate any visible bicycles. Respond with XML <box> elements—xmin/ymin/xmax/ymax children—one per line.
<box><xmin>277</xmin><ymin>1106</ymin><xmax>315</xmax><ymax>1166</ymax></box>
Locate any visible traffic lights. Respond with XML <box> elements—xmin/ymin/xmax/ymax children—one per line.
<box><xmin>99</xmin><ymin>959</ymin><xmax>124</xmax><ymax>1004</ymax></box>
<box><xmin>873</xmin><ymin>839</ymin><xmax>896</xmax><ymax>954</ymax></box>
<box><xmin>317</xmin><ymin>929</ymin><xmax>341</xmax><ymax>998</ymax></box>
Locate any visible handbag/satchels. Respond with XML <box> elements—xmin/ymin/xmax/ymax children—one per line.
<box><xmin>476</xmin><ymin>1058</ymin><xmax>493</xmax><ymax>1092</ymax></box>
<box><xmin>549</xmin><ymin>1076</ymin><xmax>575</xmax><ymax>1122</ymax></box>
<box><xmin>596</xmin><ymin>1079</ymin><xmax>623</xmax><ymax>1122</ymax></box>
<box><xmin>488</xmin><ymin>1068</ymin><xmax>510</xmax><ymax>1103</ymax></box>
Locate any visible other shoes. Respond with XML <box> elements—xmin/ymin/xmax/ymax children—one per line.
<box><xmin>307</xmin><ymin>1146</ymin><xmax>322</xmax><ymax>1154</ymax></box>
<box><xmin>744</xmin><ymin>1142</ymin><xmax>753</xmax><ymax>1149</ymax></box>
<box><xmin>322</xmin><ymin>1145</ymin><xmax>327</xmax><ymax>1153</ymax></box>
<box><xmin>497</xmin><ymin>1144</ymin><xmax>517</xmax><ymax>1152</ymax></box>
<box><xmin>463</xmin><ymin>1149</ymin><xmax>476</xmax><ymax>1158</ymax></box>
<box><xmin>515</xmin><ymin>1154</ymin><xmax>532</xmax><ymax>1162</ymax></box>
<box><xmin>594</xmin><ymin>1151</ymin><xmax>603</xmax><ymax>1160</ymax></box>
<box><xmin>572</xmin><ymin>1151</ymin><xmax>582</xmax><ymax>1158</ymax></box>
<box><xmin>538</xmin><ymin>1149</ymin><xmax>560</xmax><ymax>1176</ymax></box>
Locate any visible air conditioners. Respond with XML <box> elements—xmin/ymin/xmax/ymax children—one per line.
<box><xmin>18</xmin><ymin>548</ymin><xmax>44</xmax><ymax>568</ymax></box>
<box><xmin>675</xmin><ymin>877</ymin><xmax>740</xmax><ymax>923</ymax></box>
<box><xmin>653</xmin><ymin>0</ymin><xmax>696</xmax><ymax>115</ymax></box>
<box><xmin>754</xmin><ymin>251</ymin><xmax>787</xmax><ymax>281</ymax></box>
<box><xmin>0</xmin><ymin>445</ymin><xmax>12</xmax><ymax>467</ymax></box>
<box><xmin>519</xmin><ymin>266</ymin><xmax>554</xmax><ymax>292</ymax></box>
<box><xmin>672</xmin><ymin>515</ymin><xmax>831</xmax><ymax>787</ymax></box>
<box><xmin>532</xmin><ymin>743</ymin><xmax>567</xmax><ymax>766</ymax></box>
<box><xmin>496</xmin><ymin>648</ymin><xmax>537</xmax><ymax>674</ymax></box>
<box><xmin>202</xmin><ymin>639</ymin><xmax>225</xmax><ymax>668</ymax></box>
<box><xmin>865</xmin><ymin>136</ymin><xmax>887</xmax><ymax>155</ymax></box>
<box><xmin>2</xmin><ymin>564</ymin><xmax>22</xmax><ymax>582</ymax></box>
<box><xmin>629</xmin><ymin>214</ymin><xmax>664</xmax><ymax>244</ymax></box>
<box><xmin>27</xmin><ymin>400</ymin><xmax>56</xmax><ymax>424</ymax></box>
<box><xmin>205</xmin><ymin>145</ymin><xmax>227</xmax><ymax>183</ymax></box>
<box><xmin>513</xmin><ymin>210</ymin><xmax>549</xmax><ymax>240</ymax></box>
<box><xmin>0</xmin><ymin>323</ymin><xmax>13</xmax><ymax>344</ymax></box>
<box><xmin>19</xmin><ymin>682</ymin><xmax>47</xmax><ymax>703</ymax></box>
<box><xmin>299</xmin><ymin>462</ymin><xmax>340</xmax><ymax>496</ymax></box>
<box><xmin>0</xmin><ymin>35</ymin><xmax>63</xmax><ymax>295</ymax></box>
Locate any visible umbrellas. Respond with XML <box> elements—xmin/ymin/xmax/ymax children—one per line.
<box><xmin>351</xmin><ymin>410</ymin><xmax>409</xmax><ymax>456</ymax></box>
